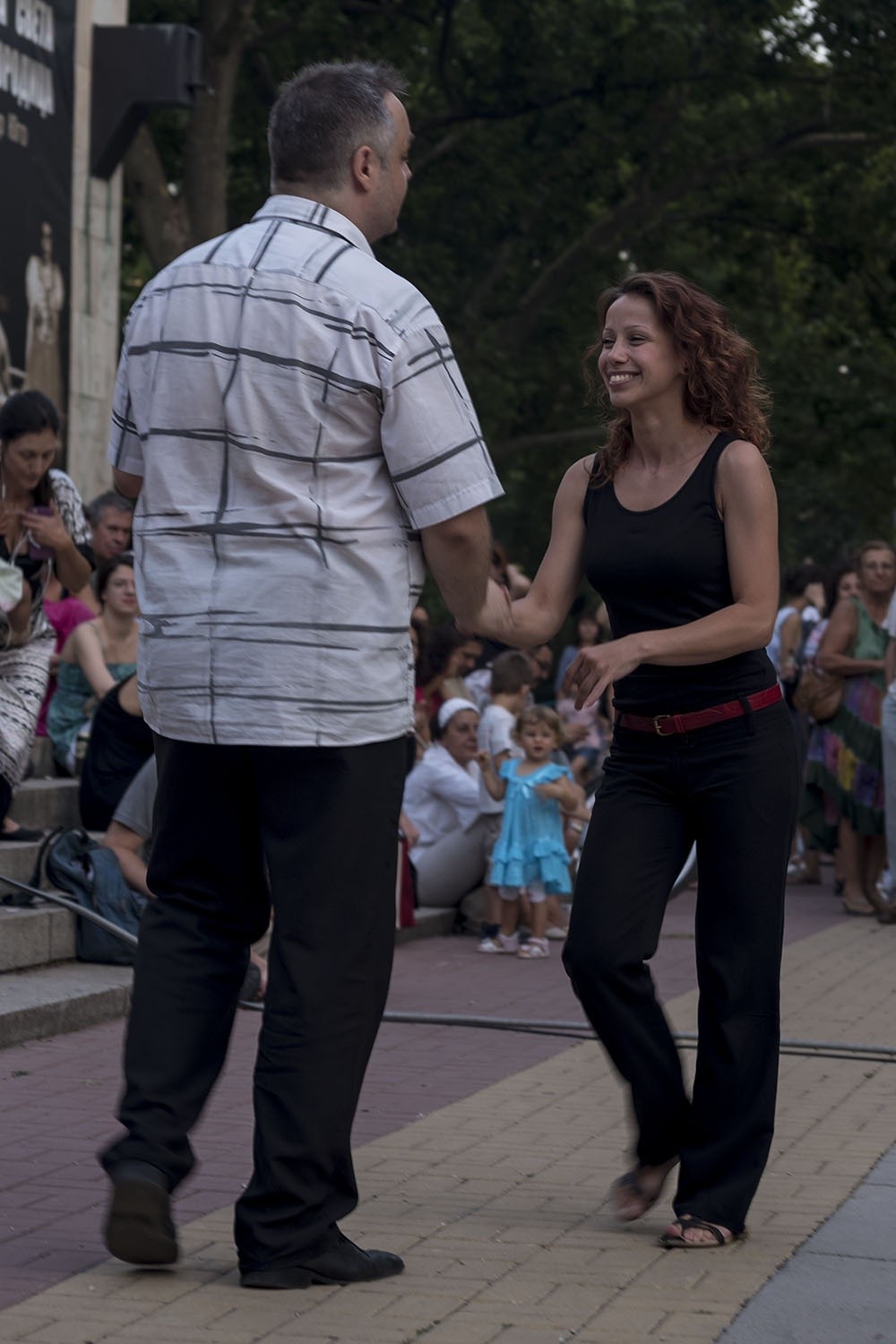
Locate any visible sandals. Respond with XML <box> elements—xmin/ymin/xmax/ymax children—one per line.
<box><xmin>515</xmin><ymin>938</ymin><xmax>548</xmax><ymax>958</ymax></box>
<box><xmin>615</xmin><ymin>1155</ymin><xmax>679</xmax><ymax>1223</ymax></box>
<box><xmin>478</xmin><ymin>933</ymin><xmax>518</xmax><ymax>952</ymax></box>
<box><xmin>659</xmin><ymin>1216</ymin><xmax>746</xmax><ymax>1248</ymax></box>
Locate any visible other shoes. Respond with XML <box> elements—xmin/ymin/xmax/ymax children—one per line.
<box><xmin>105</xmin><ymin>1161</ymin><xmax>177</xmax><ymax>1264</ymax></box>
<box><xmin>240</xmin><ymin>1224</ymin><xmax>404</xmax><ymax>1289</ymax></box>
<box><xmin>521</xmin><ymin>924</ymin><xmax>567</xmax><ymax>938</ymax></box>
<box><xmin>842</xmin><ymin>898</ymin><xmax>874</xmax><ymax>915</ymax></box>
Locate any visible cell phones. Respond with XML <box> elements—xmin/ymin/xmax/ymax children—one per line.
<box><xmin>27</xmin><ymin>508</ymin><xmax>57</xmax><ymax>561</ymax></box>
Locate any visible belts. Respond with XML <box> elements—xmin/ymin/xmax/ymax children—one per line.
<box><xmin>615</xmin><ymin>682</ymin><xmax>784</xmax><ymax>736</ymax></box>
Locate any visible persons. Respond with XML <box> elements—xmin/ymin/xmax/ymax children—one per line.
<box><xmin>22</xmin><ymin>224</ymin><xmax>65</xmax><ymax>449</ymax></box>
<box><xmin>507</xmin><ymin>269</ymin><xmax>806</xmax><ymax>1250</ymax></box>
<box><xmin>95</xmin><ymin>63</ymin><xmax>513</xmax><ymax>1289</ymax></box>
<box><xmin>0</xmin><ymin>326</ymin><xmax>896</xmax><ymax>959</ymax></box>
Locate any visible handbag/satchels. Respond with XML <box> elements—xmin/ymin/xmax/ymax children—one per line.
<box><xmin>792</xmin><ymin>657</ymin><xmax>844</xmax><ymax>720</ymax></box>
<box><xmin>22</xmin><ymin>824</ymin><xmax>144</xmax><ymax>967</ymax></box>
